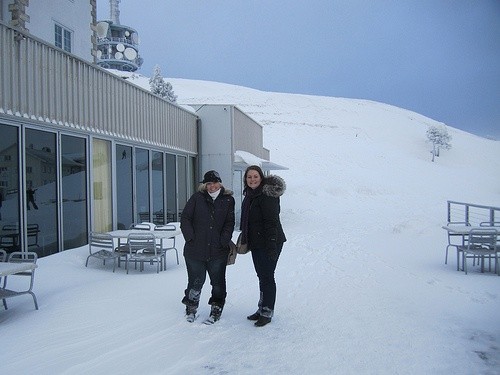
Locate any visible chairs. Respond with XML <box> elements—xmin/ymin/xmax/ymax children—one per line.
<box><xmin>0</xmin><ymin>250</ymin><xmax>39</xmax><ymax>311</ymax></box>
<box><xmin>85</xmin><ymin>223</ymin><xmax>179</xmax><ymax>274</ymax></box>
<box><xmin>0</xmin><ymin>224</ymin><xmax>40</xmax><ymax>250</ymax></box>
<box><xmin>445</xmin><ymin>222</ymin><xmax>500</xmax><ymax>276</ymax></box>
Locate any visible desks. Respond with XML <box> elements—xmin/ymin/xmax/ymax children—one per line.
<box><xmin>0</xmin><ymin>262</ymin><xmax>38</xmax><ymax>277</ymax></box>
<box><xmin>105</xmin><ymin>230</ymin><xmax>180</xmax><ymax>271</ymax></box>
<box><xmin>442</xmin><ymin>226</ymin><xmax>500</xmax><ymax>270</ymax></box>
<box><xmin>0</xmin><ymin>230</ymin><xmax>41</xmax><ymax>249</ymax></box>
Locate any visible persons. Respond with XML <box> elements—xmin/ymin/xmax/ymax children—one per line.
<box><xmin>26</xmin><ymin>185</ymin><xmax>39</xmax><ymax>210</ymax></box>
<box><xmin>180</xmin><ymin>170</ymin><xmax>235</xmax><ymax>325</ymax></box>
<box><xmin>239</xmin><ymin>165</ymin><xmax>287</xmax><ymax>327</ymax></box>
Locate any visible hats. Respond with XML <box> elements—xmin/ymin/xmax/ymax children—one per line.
<box><xmin>199</xmin><ymin>170</ymin><xmax>222</xmax><ymax>183</ymax></box>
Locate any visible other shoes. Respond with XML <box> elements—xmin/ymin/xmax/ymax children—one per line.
<box><xmin>181</xmin><ymin>297</ymin><xmax>200</xmax><ymax>322</ymax></box>
<box><xmin>247</xmin><ymin>312</ymin><xmax>260</xmax><ymax>320</ymax></box>
<box><xmin>254</xmin><ymin>317</ymin><xmax>271</xmax><ymax>327</ymax></box>
<box><xmin>203</xmin><ymin>298</ymin><xmax>224</xmax><ymax>325</ymax></box>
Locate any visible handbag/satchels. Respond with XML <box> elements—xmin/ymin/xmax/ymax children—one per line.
<box><xmin>235</xmin><ymin>232</ymin><xmax>251</xmax><ymax>255</ymax></box>
<box><xmin>227</xmin><ymin>241</ymin><xmax>237</xmax><ymax>265</ymax></box>
